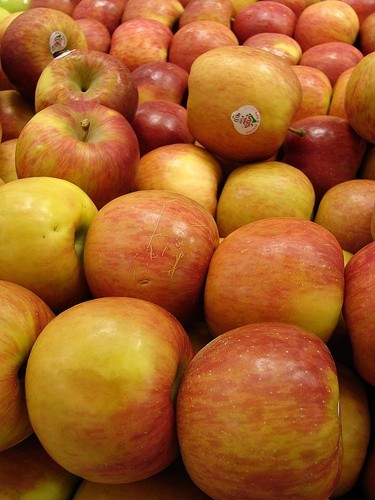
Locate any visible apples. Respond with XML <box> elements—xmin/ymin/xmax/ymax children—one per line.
<box><xmin>0</xmin><ymin>0</ymin><xmax>375</xmax><ymax>500</ymax></box>
